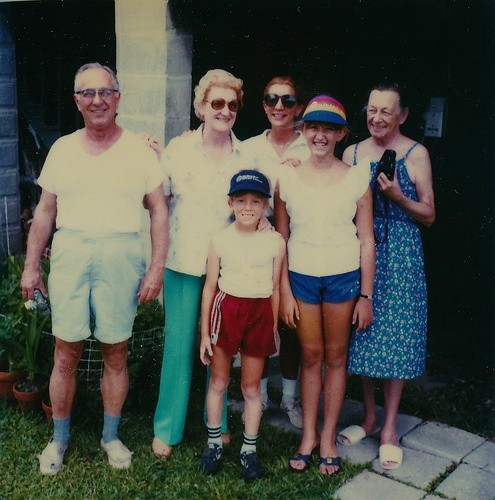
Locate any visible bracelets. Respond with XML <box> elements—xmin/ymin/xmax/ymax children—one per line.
<box><xmin>360</xmin><ymin>293</ymin><xmax>373</xmax><ymax>300</ymax></box>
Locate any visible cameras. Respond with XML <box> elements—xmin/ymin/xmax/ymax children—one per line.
<box><xmin>373</xmin><ymin>148</ymin><xmax>396</xmax><ymax>183</ymax></box>
<box><xmin>23</xmin><ymin>290</ymin><xmax>48</xmax><ymax>312</ymax></box>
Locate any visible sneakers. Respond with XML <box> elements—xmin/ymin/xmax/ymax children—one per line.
<box><xmin>280</xmin><ymin>395</ymin><xmax>305</xmax><ymax>428</ymax></box>
<box><xmin>239</xmin><ymin>449</ymin><xmax>262</xmax><ymax>478</ymax></box>
<box><xmin>200</xmin><ymin>442</ymin><xmax>224</xmax><ymax>474</ymax></box>
<box><xmin>101</xmin><ymin>437</ymin><xmax>132</xmax><ymax>467</ymax></box>
<box><xmin>241</xmin><ymin>399</ymin><xmax>269</xmax><ymax>423</ymax></box>
<box><xmin>39</xmin><ymin>436</ymin><xmax>68</xmax><ymax>475</ymax></box>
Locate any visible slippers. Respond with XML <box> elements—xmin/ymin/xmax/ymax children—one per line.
<box><xmin>337</xmin><ymin>425</ymin><xmax>382</xmax><ymax>446</ymax></box>
<box><xmin>318</xmin><ymin>458</ymin><xmax>340</xmax><ymax>476</ymax></box>
<box><xmin>288</xmin><ymin>445</ymin><xmax>316</xmax><ymax>472</ymax></box>
<box><xmin>379</xmin><ymin>443</ymin><xmax>403</xmax><ymax>469</ymax></box>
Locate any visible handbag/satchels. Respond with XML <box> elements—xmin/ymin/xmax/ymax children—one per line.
<box><xmin>375</xmin><ymin>148</ymin><xmax>396</xmax><ymax>200</ymax></box>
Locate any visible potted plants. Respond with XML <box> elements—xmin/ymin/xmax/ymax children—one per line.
<box><xmin>0</xmin><ymin>254</ymin><xmax>54</xmax><ymax>427</ymax></box>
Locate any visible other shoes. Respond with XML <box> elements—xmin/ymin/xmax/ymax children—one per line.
<box><xmin>152</xmin><ymin>436</ymin><xmax>171</xmax><ymax>458</ymax></box>
<box><xmin>223</xmin><ymin>432</ymin><xmax>231</xmax><ymax>445</ymax></box>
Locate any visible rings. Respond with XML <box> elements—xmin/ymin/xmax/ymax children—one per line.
<box><xmin>24</xmin><ymin>291</ymin><xmax>27</xmax><ymax>294</ymax></box>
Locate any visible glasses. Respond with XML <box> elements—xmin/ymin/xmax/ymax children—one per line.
<box><xmin>203</xmin><ymin>98</ymin><xmax>242</xmax><ymax>112</ymax></box>
<box><xmin>262</xmin><ymin>93</ymin><xmax>298</xmax><ymax>108</ymax></box>
<box><xmin>75</xmin><ymin>89</ymin><xmax>118</xmax><ymax>98</ymax></box>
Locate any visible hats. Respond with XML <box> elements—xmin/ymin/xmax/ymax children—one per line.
<box><xmin>227</xmin><ymin>170</ymin><xmax>271</xmax><ymax>199</ymax></box>
<box><xmin>302</xmin><ymin>94</ymin><xmax>347</xmax><ymax>125</ymax></box>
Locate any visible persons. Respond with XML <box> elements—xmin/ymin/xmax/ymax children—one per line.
<box><xmin>274</xmin><ymin>95</ymin><xmax>375</xmax><ymax>475</ymax></box>
<box><xmin>238</xmin><ymin>76</ymin><xmax>311</xmax><ymax>429</ymax></box>
<box><xmin>144</xmin><ymin>69</ymin><xmax>251</xmax><ymax>457</ymax></box>
<box><xmin>20</xmin><ymin>63</ymin><xmax>170</xmax><ymax>476</ymax></box>
<box><xmin>336</xmin><ymin>81</ymin><xmax>436</xmax><ymax>470</ymax></box>
<box><xmin>200</xmin><ymin>168</ymin><xmax>286</xmax><ymax>478</ymax></box>
